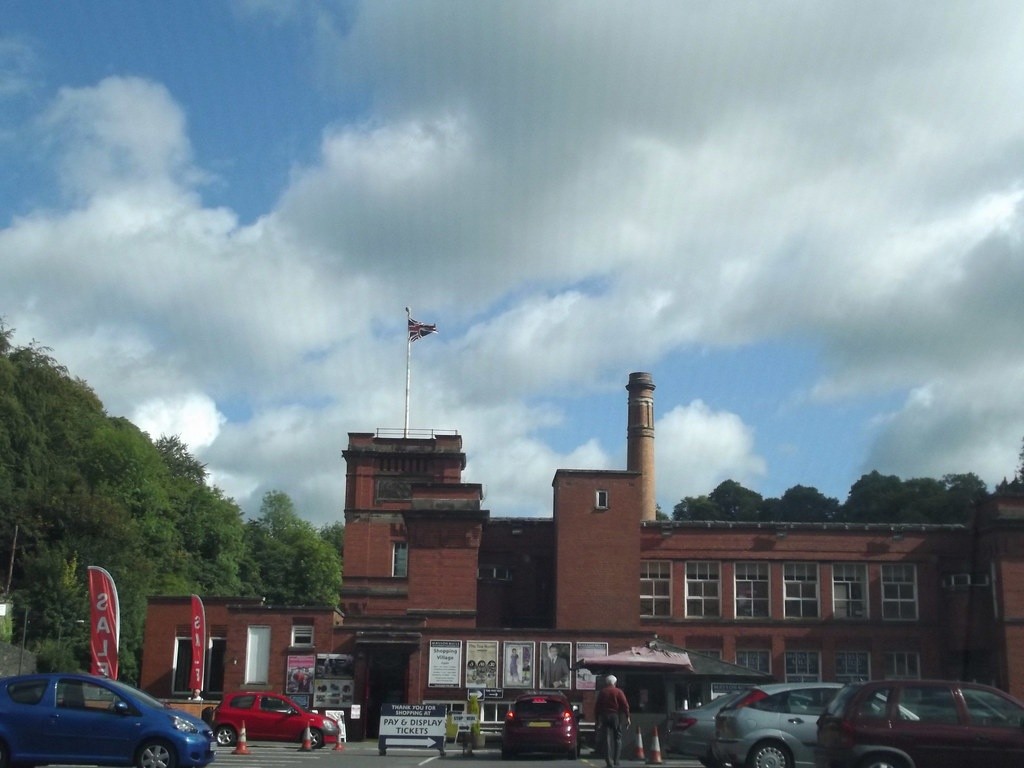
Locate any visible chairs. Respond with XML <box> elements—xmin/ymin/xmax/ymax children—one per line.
<box><xmin>917</xmin><ymin>699</ymin><xmax>957</xmax><ymax>723</ymax></box>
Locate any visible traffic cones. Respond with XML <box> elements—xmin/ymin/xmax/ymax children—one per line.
<box><xmin>231</xmin><ymin>721</ymin><xmax>252</xmax><ymax>755</ymax></box>
<box><xmin>331</xmin><ymin>731</ymin><xmax>343</xmax><ymax>750</ymax></box>
<box><xmin>297</xmin><ymin>722</ymin><xmax>315</xmax><ymax>752</ymax></box>
<box><xmin>631</xmin><ymin>725</ymin><xmax>646</xmax><ymax>761</ymax></box>
<box><xmin>647</xmin><ymin>723</ymin><xmax>664</xmax><ymax>765</ymax></box>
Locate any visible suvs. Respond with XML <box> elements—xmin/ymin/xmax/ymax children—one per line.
<box><xmin>501</xmin><ymin>692</ymin><xmax>585</xmax><ymax>760</ymax></box>
<box><xmin>201</xmin><ymin>690</ymin><xmax>342</xmax><ymax>750</ymax></box>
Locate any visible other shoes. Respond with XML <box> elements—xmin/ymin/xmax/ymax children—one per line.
<box><xmin>615</xmin><ymin>761</ymin><xmax>620</xmax><ymax>766</ymax></box>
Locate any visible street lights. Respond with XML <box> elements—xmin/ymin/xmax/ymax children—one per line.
<box><xmin>56</xmin><ymin>619</ymin><xmax>85</xmax><ymax>646</ymax></box>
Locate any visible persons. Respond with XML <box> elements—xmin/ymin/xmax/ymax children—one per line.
<box><xmin>290</xmin><ymin>666</ymin><xmax>313</xmax><ymax>693</ymax></box>
<box><xmin>188</xmin><ymin>689</ymin><xmax>203</xmax><ymax>700</ymax></box>
<box><xmin>593</xmin><ymin>674</ymin><xmax>631</xmax><ymax>768</ymax></box>
<box><xmin>508</xmin><ymin>647</ymin><xmax>522</xmax><ymax>683</ymax></box>
<box><xmin>544</xmin><ymin>646</ymin><xmax>569</xmax><ymax>688</ymax></box>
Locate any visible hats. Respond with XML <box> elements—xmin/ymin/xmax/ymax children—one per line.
<box><xmin>605</xmin><ymin>675</ymin><xmax>617</xmax><ymax>685</ymax></box>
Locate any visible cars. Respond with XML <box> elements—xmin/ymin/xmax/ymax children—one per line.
<box><xmin>715</xmin><ymin>681</ymin><xmax>920</xmax><ymax>768</ymax></box>
<box><xmin>666</xmin><ymin>691</ymin><xmax>813</xmax><ymax>767</ymax></box>
<box><xmin>814</xmin><ymin>677</ymin><xmax>1023</xmax><ymax>768</ymax></box>
<box><xmin>1</xmin><ymin>674</ymin><xmax>217</xmax><ymax>768</ymax></box>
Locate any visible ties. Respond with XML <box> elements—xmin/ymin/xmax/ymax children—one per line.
<box><xmin>551</xmin><ymin>658</ymin><xmax>554</xmax><ymax>670</ymax></box>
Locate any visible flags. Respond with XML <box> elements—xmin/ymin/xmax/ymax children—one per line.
<box><xmin>408</xmin><ymin>318</ymin><xmax>437</xmax><ymax>342</ymax></box>
